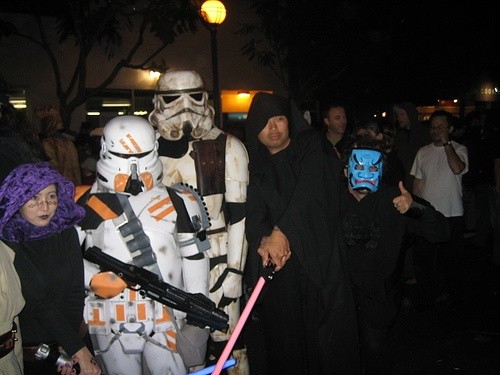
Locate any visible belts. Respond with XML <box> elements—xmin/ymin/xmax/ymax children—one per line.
<box><xmin>23</xmin><ymin>343</ymin><xmax>57</xmax><ymax>361</ymax></box>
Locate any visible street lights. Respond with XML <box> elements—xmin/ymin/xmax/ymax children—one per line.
<box><xmin>200</xmin><ymin>0</ymin><xmax>228</xmax><ymax>130</ymax></box>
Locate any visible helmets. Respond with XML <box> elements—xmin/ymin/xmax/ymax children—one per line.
<box><xmin>95</xmin><ymin>115</ymin><xmax>163</xmax><ymax>195</ymax></box>
<box><xmin>150</xmin><ymin>69</ymin><xmax>216</xmax><ymax>141</ymax></box>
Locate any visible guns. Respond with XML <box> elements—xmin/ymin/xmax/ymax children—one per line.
<box><xmin>84</xmin><ymin>245</ymin><xmax>229</xmax><ymax>335</ymax></box>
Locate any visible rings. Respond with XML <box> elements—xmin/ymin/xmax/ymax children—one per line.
<box><xmin>396</xmin><ymin>203</ymin><xmax>399</xmax><ymax>207</ymax></box>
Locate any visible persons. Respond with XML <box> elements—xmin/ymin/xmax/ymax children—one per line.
<box><xmin>245</xmin><ymin>91</ymin><xmax>359</xmax><ymax>375</ymax></box>
<box><xmin>149</xmin><ymin>71</ymin><xmax>250</xmax><ymax>375</ymax></box>
<box><xmin>0</xmin><ymin>161</ymin><xmax>103</xmax><ymax>375</ymax></box>
<box><xmin>0</xmin><ymin>70</ymin><xmax>500</xmax><ymax>375</ymax></box>
<box><xmin>0</xmin><ymin>240</ymin><xmax>26</xmax><ymax>375</ymax></box>
<box><xmin>74</xmin><ymin>115</ymin><xmax>212</xmax><ymax>375</ymax></box>
